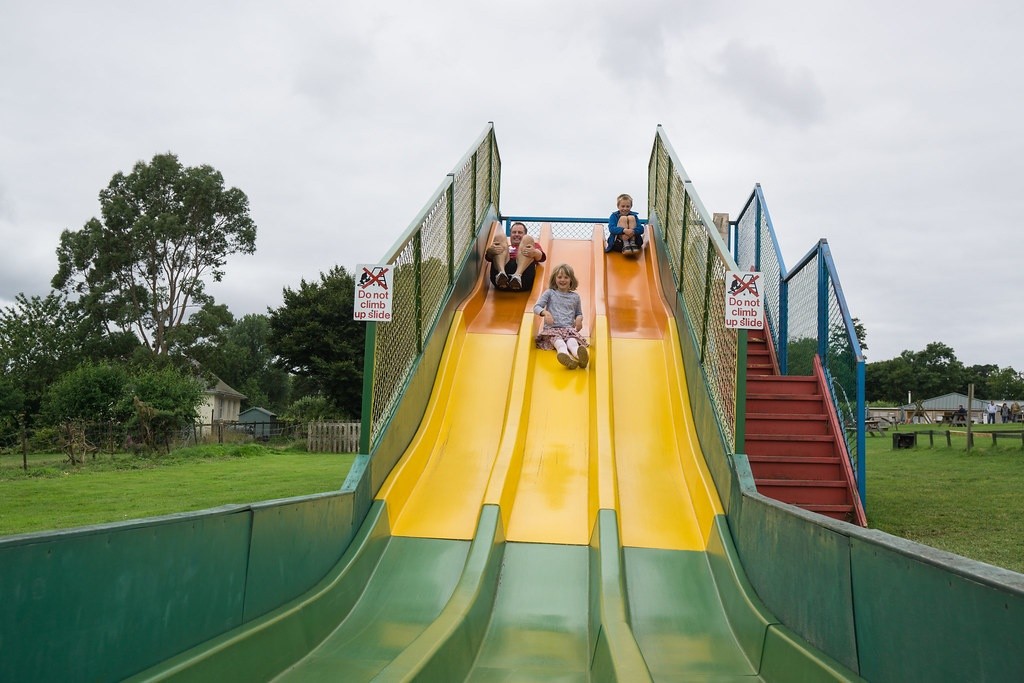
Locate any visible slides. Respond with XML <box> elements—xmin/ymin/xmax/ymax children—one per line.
<box><xmin>111</xmin><ymin>218</ymin><xmax>855</xmax><ymax>683</ymax></box>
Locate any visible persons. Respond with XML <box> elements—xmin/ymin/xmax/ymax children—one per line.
<box><xmin>485</xmin><ymin>223</ymin><xmax>546</xmax><ymax>291</ymax></box>
<box><xmin>952</xmin><ymin>401</ymin><xmax>1021</xmax><ymax>426</ymax></box>
<box><xmin>606</xmin><ymin>194</ymin><xmax>646</xmax><ymax>256</ymax></box>
<box><xmin>532</xmin><ymin>264</ymin><xmax>590</xmax><ymax>370</ymax></box>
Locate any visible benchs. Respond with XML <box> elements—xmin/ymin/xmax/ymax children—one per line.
<box><xmin>846</xmin><ymin>427</ymin><xmax>857</xmax><ymax>432</ymax></box>
<box><xmin>872</xmin><ymin>427</ymin><xmax>889</xmax><ymax>431</ymax></box>
<box><xmin>935</xmin><ymin>420</ymin><xmax>975</xmax><ymax>424</ymax></box>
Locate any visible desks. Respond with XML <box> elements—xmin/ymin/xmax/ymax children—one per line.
<box><xmin>854</xmin><ymin>420</ymin><xmax>887</xmax><ymax>437</ymax></box>
<box><xmin>943</xmin><ymin>412</ymin><xmax>967</xmax><ymax>427</ymax></box>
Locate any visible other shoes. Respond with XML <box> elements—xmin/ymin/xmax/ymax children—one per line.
<box><xmin>509</xmin><ymin>274</ymin><xmax>523</xmax><ymax>289</ymax></box>
<box><xmin>495</xmin><ymin>271</ymin><xmax>509</xmax><ymax>289</ymax></box>
<box><xmin>557</xmin><ymin>353</ymin><xmax>578</xmax><ymax>370</ymax></box>
<box><xmin>631</xmin><ymin>244</ymin><xmax>639</xmax><ymax>253</ymax></box>
<box><xmin>622</xmin><ymin>245</ymin><xmax>632</xmax><ymax>255</ymax></box>
<box><xmin>577</xmin><ymin>345</ymin><xmax>589</xmax><ymax>369</ymax></box>
